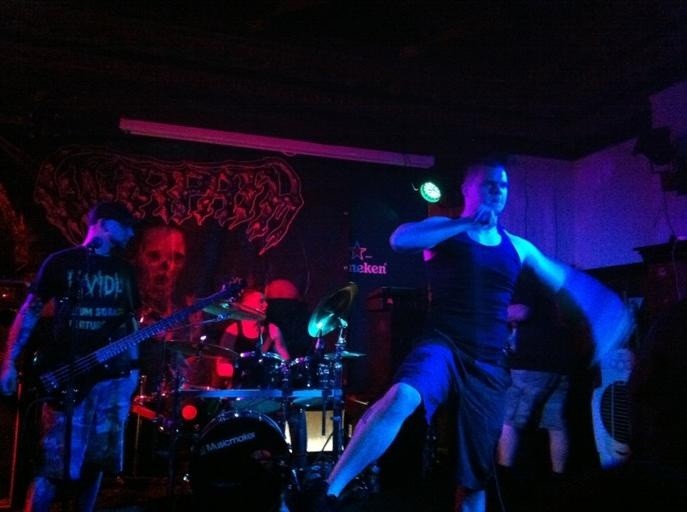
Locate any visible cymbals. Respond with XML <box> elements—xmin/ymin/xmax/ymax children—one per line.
<box><xmin>163</xmin><ymin>337</ymin><xmax>239</xmax><ymax>362</ymax></box>
<box><xmin>194</xmin><ymin>295</ymin><xmax>260</xmax><ymax>321</ymax></box>
<box><xmin>306</xmin><ymin>280</ymin><xmax>360</xmax><ymax>338</ymax></box>
<box><xmin>323</xmin><ymin>350</ymin><xmax>366</xmax><ymax>363</ymax></box>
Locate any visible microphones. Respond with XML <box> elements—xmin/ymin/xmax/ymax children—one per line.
<box><xmin>81</xmin><ymin>245</ymin><xmax>96</xmax><ymax>272</ymax></box>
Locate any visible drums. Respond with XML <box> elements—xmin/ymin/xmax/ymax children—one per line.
<box><xmin>183</xmin><ymin>408</ymin><xmax>291</xmax><ymax>508</ymax></box>
<box><xmin>233</xmin><ymin>350</ymin><xmax>287</xmax><ymax>416</ymax></box>
<box><xmin>282</xmin><ymin>355</ymin><xmax>345</xmax><ymax>407</ymax></box>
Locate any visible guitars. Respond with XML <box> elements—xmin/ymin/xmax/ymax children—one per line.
<box><xmin>23</xmin><ymin>275</ymin><xmax>248</xmax><ymax>412</ymax></box>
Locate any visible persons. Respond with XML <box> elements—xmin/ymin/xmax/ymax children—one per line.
<box><xmin>220</xmin><ymin>288</ymin><xmax>306</xmax><ymax>463</ymax></box>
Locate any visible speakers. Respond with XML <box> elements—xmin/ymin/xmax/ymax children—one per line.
<box><xmin>363</xmin><ymin>286</ymin><xmax>424</xmax><ymax>399</ymax></box>
